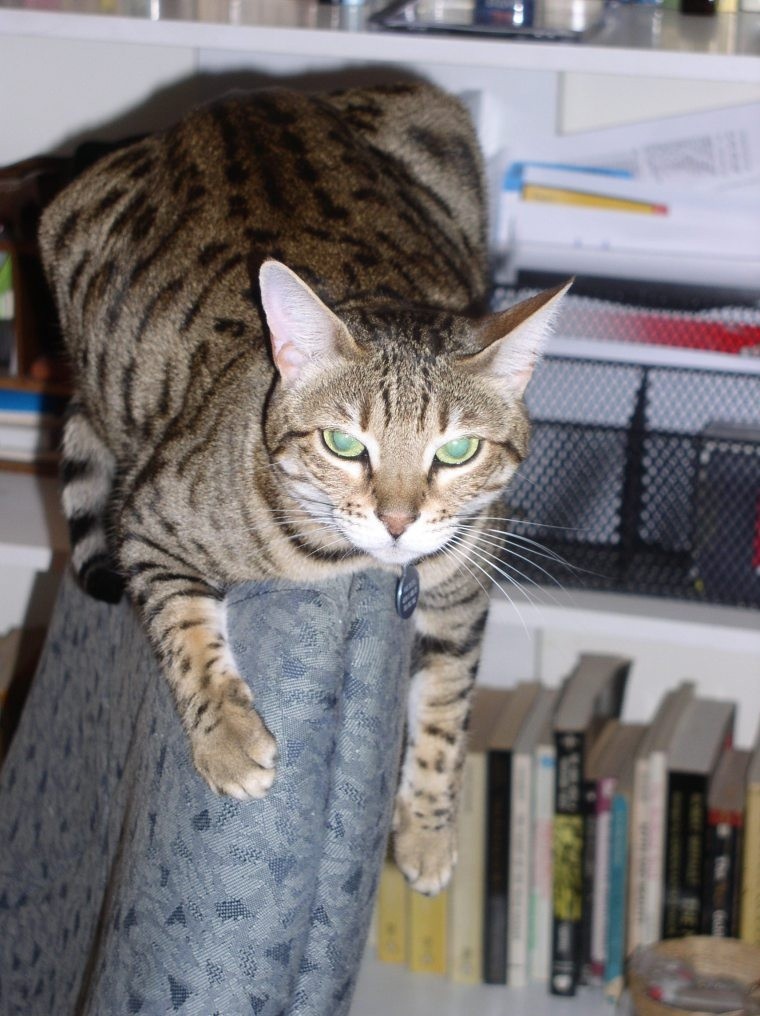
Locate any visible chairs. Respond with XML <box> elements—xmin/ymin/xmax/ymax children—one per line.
<box><xmin>0</xmin><ymin>559</ymin><xmax>417</xmax><ymax>1016</ymax></box>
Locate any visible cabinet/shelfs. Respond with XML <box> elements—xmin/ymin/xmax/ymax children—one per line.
<box><xmin>1</xmin><ymin>11</ymin><xmax>760</xmax><ymax>1014</ymax></box>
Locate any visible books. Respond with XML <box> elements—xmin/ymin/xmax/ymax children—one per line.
<box><xmin>355</xmin><ymin>647</ymin><xmax>760</xmax><ymax>1004</ymax></box>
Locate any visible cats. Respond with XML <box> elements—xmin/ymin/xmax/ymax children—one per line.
<box><xmin>37</xmin><ymin>80</ymin><xmax>574</xmax><ymax>899</ymax></box>
